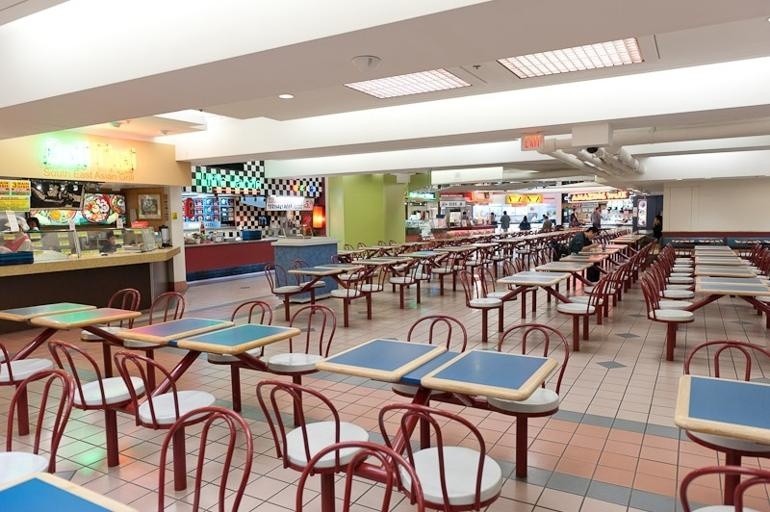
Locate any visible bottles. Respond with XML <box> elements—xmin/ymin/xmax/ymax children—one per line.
<box><xmin>160</xmin><ymin>225</ymin><xmax>169</xmax><ymax>244</ymax></box>
<box><xmin>124</xmin><ymin>228</ymin><xmax>137</xmax><ymax>246</ymax></box>
<box><xmin>200</xmin><ymin>222</ymin><xmax>206</xmax><ymax>235</ymax></box>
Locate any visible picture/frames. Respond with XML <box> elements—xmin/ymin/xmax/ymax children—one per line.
<box><xmin>136</xmin><ymin>192</ymin><xmax>163</xmax><ymax>220</ymax></box>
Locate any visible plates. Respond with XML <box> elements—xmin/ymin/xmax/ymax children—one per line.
<box><xmin>45</xmin><ymin>209</ymin><xmax>78</xmax><ymax>222</ymax></box>
<box><xmin>111</xmin><ymin>194</ymin><xmax>125</xmax><ymax>214</ymax></box>
<box><xmin>82</xmin><ymin>196</ymin><xmax>111</xmax><ymax>223</ymax></box>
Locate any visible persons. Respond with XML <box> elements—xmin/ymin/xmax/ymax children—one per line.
<box><xmin>570</xmin><ymin>213</ymin><xmax>578</xmax><ymax>227</ymax></box>
<box><xmin>542</xmin><ymin>214</ymin><xmax>552</xmax><ymax>232</ymax></box>
<box><xmin>570</xmin><ymin>226</ymin><xmax>601</xmax><ymax>283</ymax></box>
<box><xmin>27</xmin><ymin>217</ymin><xmax>40</xmax><ymax>231</ymax></box>
<box><xmin>462</xmin><ymin>212</ymin><xmax>469</xmax><ymax>226</ymax></box>
<box><xmin>519</xmin><ymin>216</ymin><xmax>530</xmax><ymax>230</ymax></box>
<box><xmin>489</xmin><ymin>211</ymin><xmax>496</xmax><ymax>225</ymax></box>
<box><xmin>590</xmin><ymin>207</ymin><xmax>606</xmax><ymax>240</ymax></box>
<box><xmin>638</xmin><ymin>215</ymin><xmax>662</xmax><ymax>250</ymax></box>
<box><xmin>100</xmin><ymin>231</ymin><xmax>121</xmax><ymax>253</ymax></box>
<box><xmin>499</xmin><ymin>211</ymin><xmax>510</xmax><ymax>232</ymax></box>
<box><xmin>607</xmin><ymin>207</ymin><xmax>624</xmax><ymax>212</ymax></box>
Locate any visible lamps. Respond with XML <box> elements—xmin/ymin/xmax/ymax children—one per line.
<box><xmin>312</xmin><ymin>205</ymin><xmax>324</xmax><ymax>228</ymax></box>
